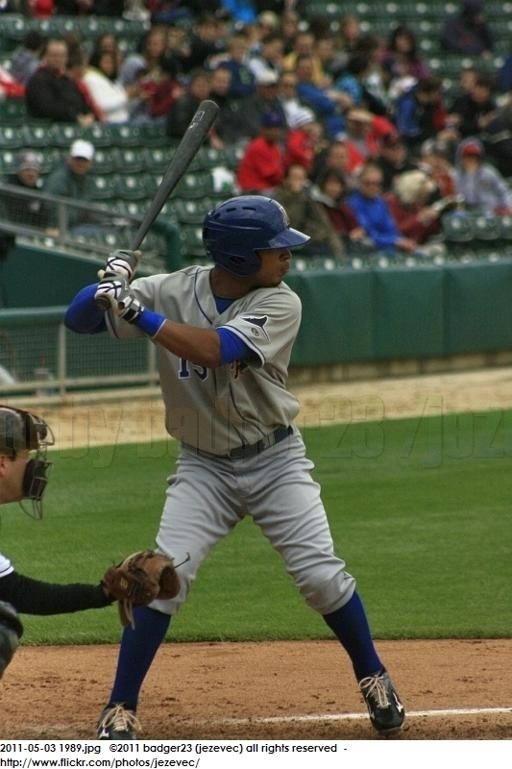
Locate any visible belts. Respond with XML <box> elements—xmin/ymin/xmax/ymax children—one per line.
<box><xmin>180</xmin><ymin>424</ymin><xmax>294</xmax><ymax>462</ymax></box>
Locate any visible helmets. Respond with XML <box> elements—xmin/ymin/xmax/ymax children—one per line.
<box><xmin>0</xmin><ymin>406</ymin><xmax>53</xmax><ymax>456</ymax></box>
<box><xmin>201</xmin><ymin>193</ymin><xmax>313</xmax><ymax>280</ymax></box>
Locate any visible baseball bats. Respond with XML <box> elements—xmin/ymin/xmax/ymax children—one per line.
<box><xmin>92</xmin><ymin>100</ymin><xmax>222</xmax><ymax>312</ymax></box>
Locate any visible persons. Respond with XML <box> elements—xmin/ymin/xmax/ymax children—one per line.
<box><xmin>63</xmin><ymin>195</ymin><xmax>407</xmax><ymax>738</ymax></box>
<box><xmin>0</xmin><ymin>405</ymin><xmax>181</xmax><ymax>684</ymax></box>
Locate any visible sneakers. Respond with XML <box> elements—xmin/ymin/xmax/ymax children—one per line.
<box><xmin>94</xmin><ymin>702</ymin><xmax>137</xmax><ymax>740</ymax></box>
<box><xmin>352</xmin><ymin>660</ymin><xmax>406</xmax><ymax>734</ymax></box>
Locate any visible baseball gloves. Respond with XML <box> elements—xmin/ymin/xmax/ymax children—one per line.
<box><xmin>101</xmin><ymin>550</ymin><xmax>181</xmax><ymax>625</ymax></box>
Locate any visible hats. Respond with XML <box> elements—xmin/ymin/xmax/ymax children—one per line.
<box><xmin>260</xmin><ymin>112</ymin><xmax>283</xmax><ymax>127</ymax></box>
<box><xmin>70</xmin><ymin>138</ymin><xmax>95</xmax><ymax>163</ymax></box>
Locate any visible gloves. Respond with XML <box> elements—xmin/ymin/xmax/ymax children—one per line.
<box><xmin>104</xmin><ymin>249</ymin><xmax>142</xmax><ymax>284</ymax></box>
<box><xmin>93</xmin><ymin>268</ymin><xmax>146</xmax><ymax>325</ymax></box>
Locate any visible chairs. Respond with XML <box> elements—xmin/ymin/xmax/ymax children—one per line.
<box><xmin>2</xmin><ymin>0</ymin><xmax>512</xmax><ymax>277</ymax></box>
